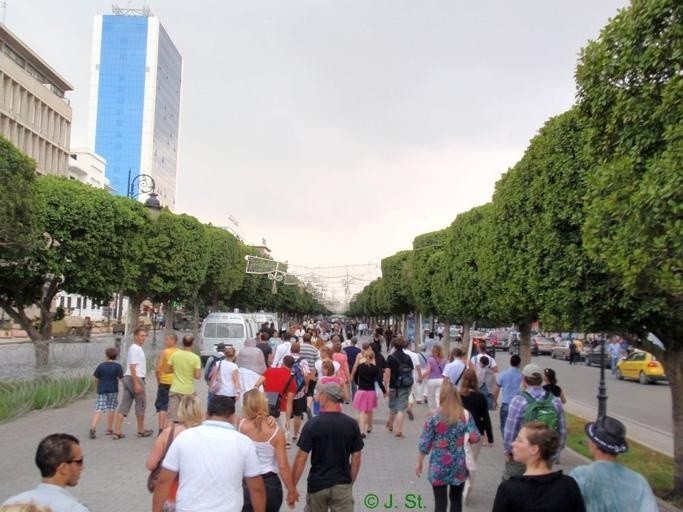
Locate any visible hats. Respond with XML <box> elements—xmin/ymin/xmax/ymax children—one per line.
<box><xmin>521</xmin><ymin>363</ymin><xmax>542</xmax><ymax>377</ymax></box>
<box><xmin>315</xmin><ymin>382</ymin><xmax>344</xmax><ymax>402</ymax></box>
<box><xmin>216</xmin><ymin>343</ymin><xmax>227</xmax><ymax>351</ymax></box>
<box><xmin>584</xmin><ymin>414</ymin><xmax>628</xmax><ymax>453</ymax></box>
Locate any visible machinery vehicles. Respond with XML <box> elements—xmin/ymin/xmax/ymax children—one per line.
<box><xmin>110</xmin><ymin>315</ymin><xmax>152</xmax><ymax>336</ymax></box>
<box><xmin>36</xmin><ymin>310</ymin><xmax>87</xmax><ymax>339</ymax></box>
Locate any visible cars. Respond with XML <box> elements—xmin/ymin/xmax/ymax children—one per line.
<box><xmin>530</xmin><ymin>336</ymin><xmax>556</xmax><ymax>355</ymax></box>
<box><xmin>429</xmin><ymin>325</ymin><xmax>522</xmax><ymax>358</ymax></box>
<box><xmin>585</xmin><ymin>342</ymin><xmax>619</xmax><ymax>368</ymax></box>
<box><xmin>616</xmin><ymin>350</ymin><xmax>668</xmax><ymax>384</ymax></box>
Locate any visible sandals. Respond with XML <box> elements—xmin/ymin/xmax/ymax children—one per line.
<box><xmin>361</xmin><ymin>409</ymin><xmax>414</xmax><ymax>440</ymax></box>
<box><xmin>90</xmin><ymin>429</ymin><xmax>97</xmax><ymax>438</ymax></box>
<box><xmin>137</xmin><ymin>429</ymin><xmax>153</xmax><ymax>437</ymax></box>
<box><xmin>105</xmin><ymin>430</ymin><xmax>114</xmax><ymax>435</ymax></box>
<box><xmin>113</xmin><ymin>433</ymin><xmax>126</xmax><ymax>440</ymax></box>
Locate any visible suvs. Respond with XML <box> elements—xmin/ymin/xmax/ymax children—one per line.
<box><xmin>551</xmin><ymin>339</ymin><xmax>583</xmax><ymax>361</ymax></box>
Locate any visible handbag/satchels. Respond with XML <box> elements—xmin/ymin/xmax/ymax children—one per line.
<box><xmin>462</xmin><ymin>474</ymin><xmax>472</xmax><ymax>505</ymax></box>
<box><xmin>463</xmin><ymin>410</ymin><xmax>478</xmax><ymax>476</ymax></box>
<box><xmin>265</xmin><ymin>391</ymin><xmax>280</xmax><ymax>409</ymax></box>
<box><xmin>209</xmin><ymin>360</ymin><xmax>222</xmax><ymax>394</ymax></box>
<box><xmin>148</xmin><ymin>423</ymin><xmax>175</xmax><ymax>493</ymax></box>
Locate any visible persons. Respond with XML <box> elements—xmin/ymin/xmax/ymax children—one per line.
<box><xmin>1</xmin><ymin>318</ymin><xmax>657</xmax><ymax>511</ymax></box>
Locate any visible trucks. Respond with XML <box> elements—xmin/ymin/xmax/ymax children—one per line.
<box><xmin>175</xmin><ymin>311</ymin><xmax>200</xmax><ymax>330</ymax></box>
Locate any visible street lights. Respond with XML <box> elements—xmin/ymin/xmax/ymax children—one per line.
<box><xmin>128</xmin><ymin>169</ymin><xmax>163</xmax><ymax>222</ymax></box>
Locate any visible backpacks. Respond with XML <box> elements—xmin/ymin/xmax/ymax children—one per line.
<box><xmin>391</xmin><ymin>354</ymin><xmax>413</xmax><ymax>388</ymax></box>
<box><xmin>517</xmin><ymin>390</ymin><xmax>559</xmax><ymax>432</ymax></box>
<box><xmin>291</xmin><ymin>358</ymin><xmax>305</xmax><ymax>394</ymax></box>
<box><xmin>207</xmin><ymin>356</ymin><xmax>225</xmax><ymax>385</ymax></box>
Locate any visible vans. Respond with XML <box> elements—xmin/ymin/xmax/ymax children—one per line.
<box><xmin>199</xmin><ymin>312</ymin><xmax>258</xmax><ymax>363</ymax></box>
<box><xmin>250</xmin><ymin>312</ymin><xmax>279</xmax><ymax>337</ymax></box>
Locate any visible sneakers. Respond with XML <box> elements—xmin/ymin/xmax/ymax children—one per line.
<box><xmin>282</xmin><ymin>406</ymin><xmax>313</xmax><ymax>449</ymax></box>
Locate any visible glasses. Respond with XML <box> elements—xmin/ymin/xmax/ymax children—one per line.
<box><xmin>67</xmin><ymin>456</ymin><xmax>84</xmax><ymax>464</ymax></box>
<box><xmin>545</xmin><ymin>368</ymin><xmax>548</xmax><ymax>372</ymax></box>
